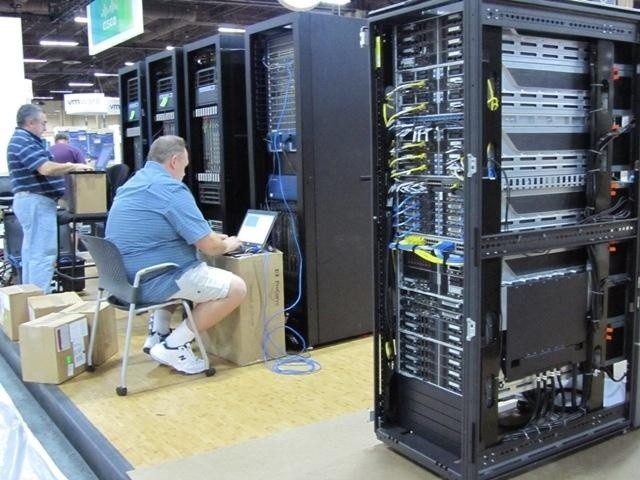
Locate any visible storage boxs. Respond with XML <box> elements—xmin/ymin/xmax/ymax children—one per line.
<box><xmin>0</xmin><ymin>284</ymin><xmax>119</xmax><ymax>385</ymax></box>
<box><xmin>192</xmin><ymin>247</ymin><xmax>287</xmax><ymax>368</ymax></box>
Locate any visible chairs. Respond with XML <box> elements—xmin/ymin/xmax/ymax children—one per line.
<box><xmin>78</xmin><ymin>234</ymin><xmax>216</xmax><ymax>395</ymax></box>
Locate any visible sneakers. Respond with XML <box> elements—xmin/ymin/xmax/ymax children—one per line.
<box><xmin>149</xmin><ymin>340</ymin><xmax>205</xmax><ymax>374</ymax></box>
<box><xmin>142</xmin><ymin>327</ymin><xmax>172</xmax><ymax>354</ymax></box>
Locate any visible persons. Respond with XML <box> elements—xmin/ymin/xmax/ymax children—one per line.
<box><xmin>6</xmin><ymin>104</ymin><xmax>94</xmax><ymax>295</ymax></box>
<box><xmin>103</xmin><ymin>133</ymin><xmax>249</xmax><ymax>376</ymax></box>
<box><xmin>48</xmin><ymin>131</ymin><xmax>86</xmax><ymax>208</ymax></box>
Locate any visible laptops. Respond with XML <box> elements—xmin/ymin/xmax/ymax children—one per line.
<box><xmin>223</xmin><ymin>209</ymin><xmax>279</xmax><ymax>257</ymax></box>
<box><xmin>66</xmin><ymin>143</ymin><xmax>114</xmax><ymax>174</ymax></box>
